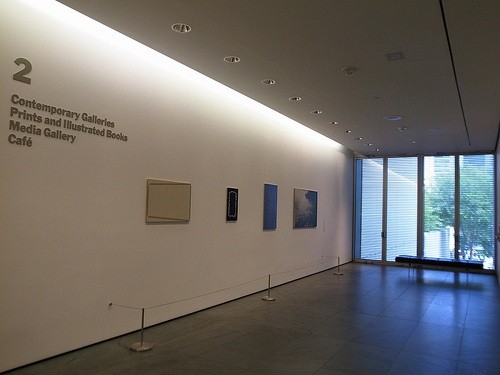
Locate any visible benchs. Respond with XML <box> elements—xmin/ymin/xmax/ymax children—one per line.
<box><xmin>395</xmin><ymin>255</ymin><xmax>483</xmax><ymax>284</ymax></box>
